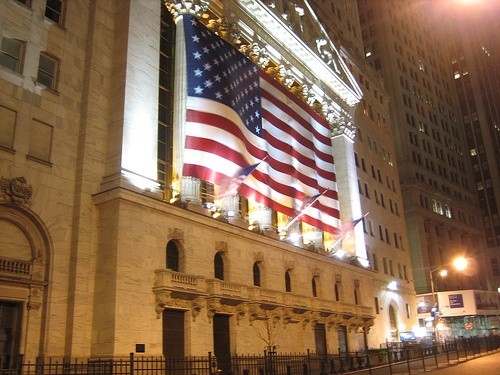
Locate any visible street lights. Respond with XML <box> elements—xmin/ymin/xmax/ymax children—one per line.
<box><xmin>429</xmin><ymin>254</ymin><xmax>470</xmax><ymax>354</ymax></box>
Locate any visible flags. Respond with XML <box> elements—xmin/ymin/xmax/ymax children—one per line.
<box><xmin>343</xmin><ymin>216</ymin><xmax>365</xmax><ymax>233</ymax></box>
<box><xmin>217</xmin><ymin>162</ymin><xmax>261</xmax><ymax>197</ymax></box>
<box><xmin>183</xmin><ymin>14</ymin><xmax>341</xmax><ymax>238</ymax></box>
<box><xmin>300</xmin><ymin>194</ymin><xmax>323</xmax><ymax>218</ymax></box>
